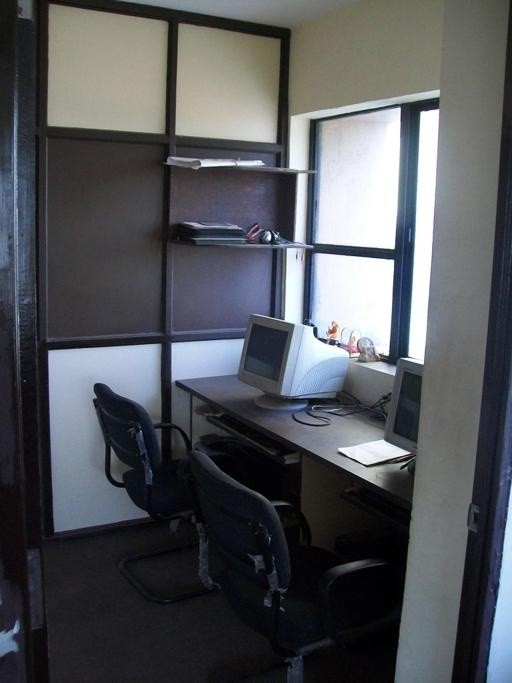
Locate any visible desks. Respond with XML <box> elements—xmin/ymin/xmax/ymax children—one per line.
<box><xmin>170</xmin><ymin>373</ymin><xmax>415</xmax><ymax>545</ymax></box>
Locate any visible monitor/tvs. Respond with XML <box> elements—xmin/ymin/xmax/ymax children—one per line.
<box><xmin>383</xmin><ymin>357</ymin><xmax>424</xmax><ymax>472</ymax></box>
<box><xmin>238</xmin><ymin>313</ymin><xmax>350</xmax><ymax>413</ymax></box>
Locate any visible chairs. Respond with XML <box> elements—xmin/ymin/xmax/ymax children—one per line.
<box><xmin>187</xmin><ymin>450</ymin><xmax>401</xmax><ymax>682</ymax></box>
<box><xmin>94</xmin><ymin>383</ymin><xmax>294</xmax><ymax>603</ymax></box>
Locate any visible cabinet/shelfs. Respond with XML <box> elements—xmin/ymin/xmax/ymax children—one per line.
<box><xmin>167</xmin><ymin>155</ymin><xmax>317</xmax><ymax>250</ymax></box>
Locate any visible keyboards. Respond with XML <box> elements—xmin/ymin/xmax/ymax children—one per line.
<box><xmin>206</xmin><ymin>413</ymin><xmax>296</xmax><ymax>456</ymax></box>
<box><xmin>343</xmin><ymin>486</ymin><xmax>409</xmax><ymax>531</ymax></box>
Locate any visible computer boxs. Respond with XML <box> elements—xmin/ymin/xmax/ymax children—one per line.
<box><xmin>193</xmin><ymin>438</ymin><xmax>302</xmax><ymax>545</ymax></box>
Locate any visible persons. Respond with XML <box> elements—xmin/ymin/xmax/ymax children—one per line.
<box><xmin>326</xmin><ymin>321</ymin><xmax>341</xmax><ymax>347</ymax></box>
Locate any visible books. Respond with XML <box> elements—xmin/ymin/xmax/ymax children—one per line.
<box><xmin>176</xmin><ymin>221</ymin><xmax>246</xmax><ymax>246</ymax></box>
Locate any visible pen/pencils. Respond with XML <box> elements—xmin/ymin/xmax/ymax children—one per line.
<box><xmin>400</xmin><ymin>456</ymin><xmax>416</xmax><ymax>470</ymax></box>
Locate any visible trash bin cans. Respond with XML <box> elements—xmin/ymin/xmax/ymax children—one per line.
<box><xmin>195</xmin><ymin>436</ymin><xmax>260</xmax><ymax>491</ymax></box>
